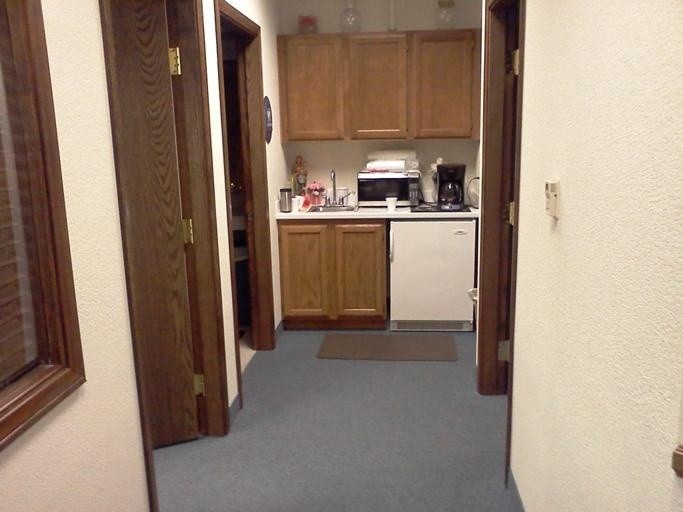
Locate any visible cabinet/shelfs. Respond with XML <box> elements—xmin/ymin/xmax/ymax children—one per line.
<box><xmin>277</xmin><ymin>28</ymin><xmax>480</xmax><ymax>147</ymax></box>
<box><xmin>277</xmin><ymin>219</ymin><xmax>388</xmax><ymax>331</ymax></box>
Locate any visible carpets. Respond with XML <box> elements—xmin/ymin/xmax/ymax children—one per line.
<box><xmin>316</xmin><ymin>332</ymin><xmax>460</xmax><ymax>361</ymax></box>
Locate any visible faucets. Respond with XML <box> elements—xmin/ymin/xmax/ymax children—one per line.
<box><xmin>329</xmin><ymin>169</ymin><xmax>339</xmax><ymax>204</ymax></box>
<box><xmin>340</xmin><ymin>190</ymin><xmax>356</xmax><ymax>205</ymax></box>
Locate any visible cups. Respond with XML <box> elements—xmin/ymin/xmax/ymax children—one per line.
<box><xmin>386</xmin><ymin>196</ymin><xmax>398</xmax><ymax>212</ymax></box>
<box><xmin>291</xmin><ymin>196</ymin><xmax>303</xmax><ymax>211</ymax></box>
<box><xmin>366</xmin><ymin>158</ymin><xmax>406</xmax><ymax>173</ymax></box>
<box><xmin>421</xmin><ymin>191</ymin><xmax>434</xmax><ymax>202</ymax></box>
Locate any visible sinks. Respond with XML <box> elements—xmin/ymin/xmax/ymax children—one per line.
<box><xmin>305</xmin><ymin>202</ymin><xmax>355</xmax><ymax>214</ymax></box>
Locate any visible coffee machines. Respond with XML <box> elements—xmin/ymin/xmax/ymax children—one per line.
<box><xmin>436</xmin><ymin>163</ymin><xmax>466</xmax><ymax>210</ymax></box>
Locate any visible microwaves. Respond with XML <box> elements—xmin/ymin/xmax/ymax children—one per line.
<box><xmin>356</xmin><ymin>169</ymin><xmax>420</xmax><ymax>207</ymax></box>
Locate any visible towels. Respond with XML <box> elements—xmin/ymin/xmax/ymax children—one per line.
<box><xmin>366</xmin><ymin>159</ymin><xmax>405</xmax><ymax>170</ymax></box>
<box><xmin>365</xmin><ymin>150</ymin><xmax>417</xmax><ymax>161</ymax></box>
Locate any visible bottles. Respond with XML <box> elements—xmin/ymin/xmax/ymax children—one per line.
<box><xmin>278</xmin><ymin>187</ymin><xmax>291</xmax><ymax>211</ymax></box>
<box><xmin>340</xmin><ymin>0</ymin><xmax>362</xmax><ymax>33</ymax></box>
<box><xmin>433</xmin><ymin>1</ymin><xmax>460</xmax><ymax>30</ymax></box>
